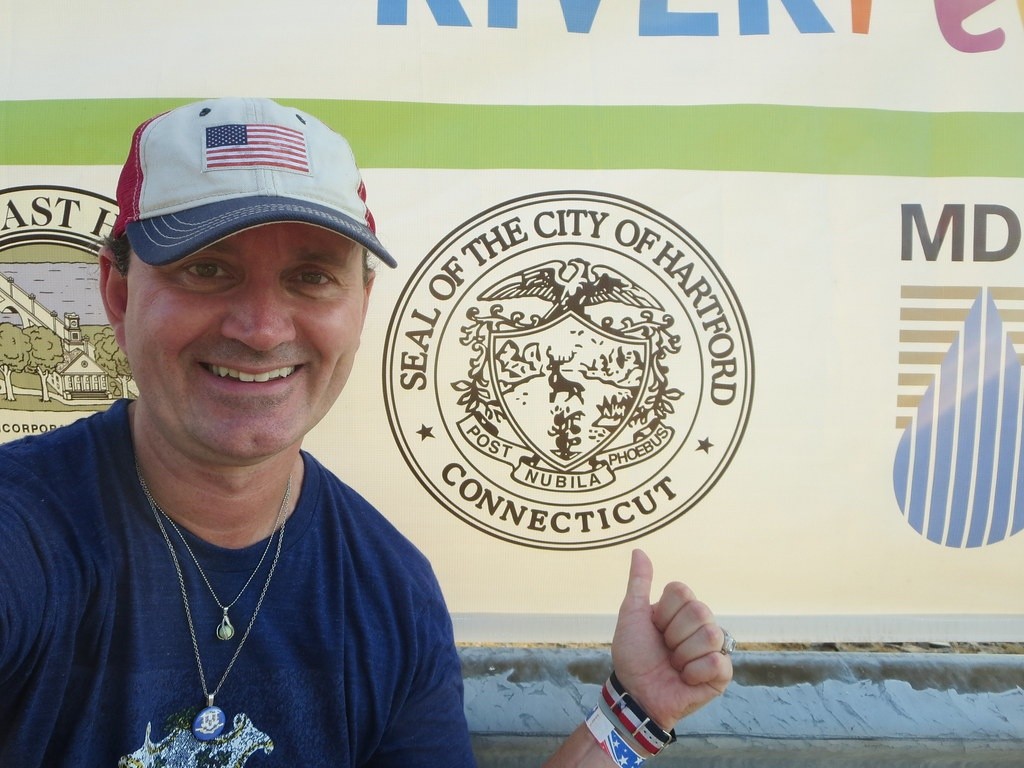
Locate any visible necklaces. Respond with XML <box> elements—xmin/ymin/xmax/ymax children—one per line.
<box><xmin>133</xmin><ymin>460</ymin><xmax>299</xmax><ymax>742</ymax></box>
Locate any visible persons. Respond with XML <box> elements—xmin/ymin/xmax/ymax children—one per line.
<box><xmin>0</xmin><ymin>96</ymin><xmax>733</xmax><ymax>768</ymax></box>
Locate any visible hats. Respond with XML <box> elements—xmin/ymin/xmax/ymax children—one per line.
<box><xmin>113</xmin><ymin>94</ymin><xmax>399</xmax><ymax>271</ymax></box>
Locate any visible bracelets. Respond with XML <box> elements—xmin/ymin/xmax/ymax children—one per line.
<box><xmin>584</xmin><ymin>670</ymin><xmax>678</xmax><ymax>768</ymax></box>
